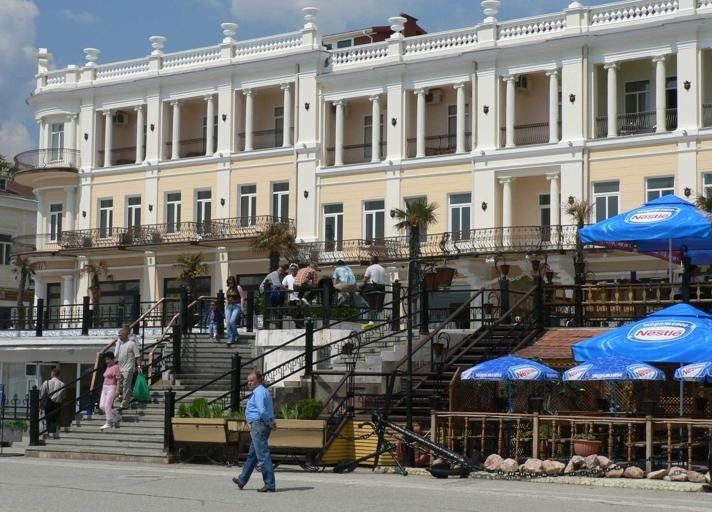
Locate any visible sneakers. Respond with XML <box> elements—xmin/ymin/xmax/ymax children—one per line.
<box><xmin>99</xmin><ymin>424</ymin><xmax>112</xmax><ymax>430</ymax></box>
<box><xmin>288</xmin><ymin>294</ymin><xmax>346</xmax><ymax>306</ymax></box>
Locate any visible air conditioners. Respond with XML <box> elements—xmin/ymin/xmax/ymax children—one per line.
<box><xmin>113</xmin><ymin>112</ymin><xmax>129</xmax><ymax>126</ymax></box>
<box><xmin>514</xmin><ymin>75</ymin><xmax>531</xmax><ymax>90</ymax></box>
<box><xmin>425</xmin><ymin>89</ymin><xmax>444</xmax><ymax>105</ymax></box>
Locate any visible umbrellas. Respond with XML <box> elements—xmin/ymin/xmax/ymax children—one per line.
<box><xmin>578</xmin><ymin>194</ymin><xmax>711</xmax><ymax>299</ymax></box>
<box><xmin>561</xmin><ymin>357</ymin><xmax>666</xmax><ymax>457</ymax></box>
<box><xmin>672</xmin><ymin>362</ymin><xmax>711</xmax><ymax>388</ymax></box>
<box><xmin>461</xmin><ymin>354</ymin><xmax>560</xmax><ymax>455</ymax></box>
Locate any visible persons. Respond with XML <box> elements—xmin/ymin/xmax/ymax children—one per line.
<box><xmin>114</xmin><ymin>324</ymin><xmax>142</xmax><ymax>359</ymax></box>
<box><xmin>570</xmin><ymin>302</ymin><xmax>712</xmax><ymax>460</ymax></box>
<box><xmin>231</xmin><ymin>371</ymin><xmax>276</xmax><ymax>491</ymax></box>
<box><xmin>114</xmin><ymin>330</ymin><xmax>144</xmax><ymax>411</ymax></box>
<box><xmin>206</xmin><ymin>256</ymin><xmax>387</xmax><ymax>345</ymax></box>
<box><xmin>98</xmin><ymin>352</ymin><xmax>121</xmax><ymax>430</ymax></box>
<box><xmin>694</xmin><ymin>384</ymin><xmax>707</xmax><ymax>416</ymax></box>
<box><xmin>39</xmin><ymin>367</ymin><xmax>66</xmax><ymax>439</ymax></box>
<box><xmin>677</xmin><ymin>255</ymin><xmax>702</xmax><ymax>275</ymax></box>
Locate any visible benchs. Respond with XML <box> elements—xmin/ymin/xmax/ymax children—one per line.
<box><xmin>546</xmin><ymin>291</ymin><xmax>652</xmax><ymax>329</ymax></box>
<box><xmin>55</xmin><ymin>223</ymin><xmax>227</xmax><ymax>249</ymax></box>
<box><xmin>445</xmin><ymin>409</ymin><xmax>712</xmax><ymax>477</ymax></box>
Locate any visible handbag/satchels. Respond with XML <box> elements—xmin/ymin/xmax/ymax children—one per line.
<box><xmin>40</xmin><ymin>379</ymin><xmax>52</xmax><ymax>411</ymax></box>
<box><xmin>133</xmin><ymin>372</ymin><xmax>151</xmax><ymax>402</ymax></box>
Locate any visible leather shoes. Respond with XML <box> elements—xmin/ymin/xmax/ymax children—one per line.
<box><xmin>232</xmin><ymin>477</ymin><xmax>243</xmax><ymax>488</ymax></box>
<box><xmin>116</xmin><ymin>407</ymin><xmax>128</xmax><ymax>411</ymax></box>
<box><xmin>258</xmin><ymin>486</ymin><xmax>275</xmax><ymax>492</ymax></box>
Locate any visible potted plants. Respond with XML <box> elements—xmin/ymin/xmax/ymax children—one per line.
<box><xmin>255</xmin><ymin>400</ymin><xmax>328</xmax><ymax>474</ymax></box>
<box><xmin>170</xmin><ymin>397</ymin><xmax>239</xmax><ymax>467</ymax></box>
